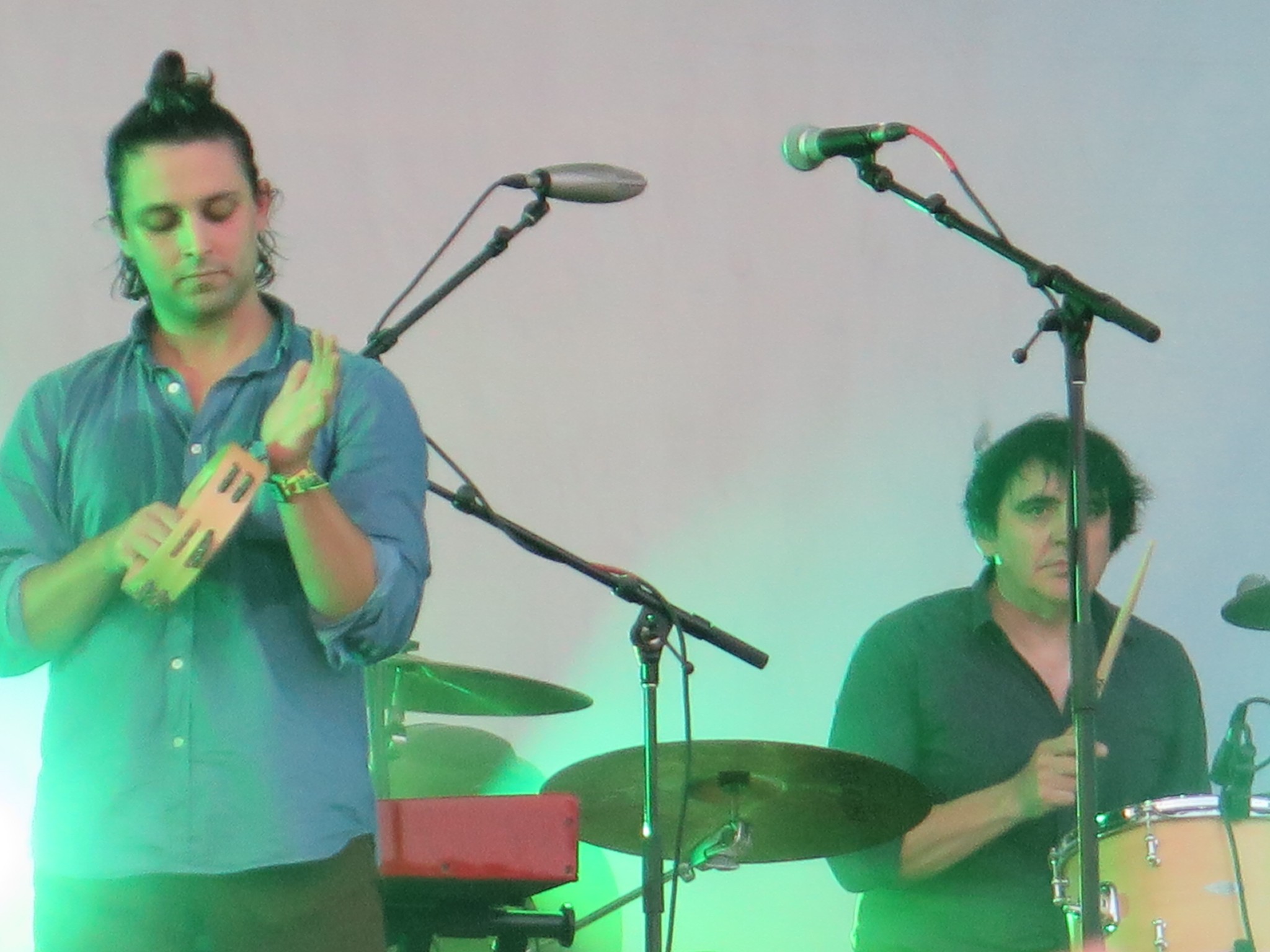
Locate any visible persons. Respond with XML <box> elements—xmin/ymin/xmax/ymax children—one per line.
<box><xmin>0</xmin><ymin>44</ymin><xmax>431</xmax><ymax>951</ymax></box>
<box><xmin>820</xmin><ymin>413</ymin><xmax>1213</xmax><ymax>951</ymax></box>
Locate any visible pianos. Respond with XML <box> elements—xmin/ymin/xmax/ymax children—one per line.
<box><xmin>373</xmin><ymin>796</ymin><xmax>578</xmax><ymax>899</ymax></box>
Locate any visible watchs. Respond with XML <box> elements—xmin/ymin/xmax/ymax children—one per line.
<box><xmin>259</xmin><ymin>462</ymin><xmax>329</xmax><ymax>501</ymax></box>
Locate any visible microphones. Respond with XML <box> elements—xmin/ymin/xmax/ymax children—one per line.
<box><xmin>1212</xmin><ymin>700</ymin><xmax>1248</xmax><ymax>787</ymax></box>
<box><xmin>496</xmin><ymin>158</ymin><xmax>642</xmax><ymax>204</ymax></box>
<box><xmin>779</xmin><ymin>123</ymin><xmax>912</xmax><ymax>171</ymax></box>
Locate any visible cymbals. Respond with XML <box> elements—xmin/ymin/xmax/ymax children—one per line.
<box><xmin>380</xmin><ymin>656</ymin><xmax>594</xmax><ymax>715</ymax></box>
<box><xmin>1224</xmin><ymin>584</ymin><xmax>1269</xmax><ymax>631</ymax></box>
<box><xmin>120</xmin><ymin>442</ymin><xmax>268</xmax><ymax>614</ymax></box>
<box><xmin>539</xmin><ymin>739</ymin><xmax>931</xmax><ymax>864</ymax></box>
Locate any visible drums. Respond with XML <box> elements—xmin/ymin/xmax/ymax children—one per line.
<box><xmin>1048</xmin><ymin>792</ymin><xmax>1269</xmax><ymax>952</ymax></box>
